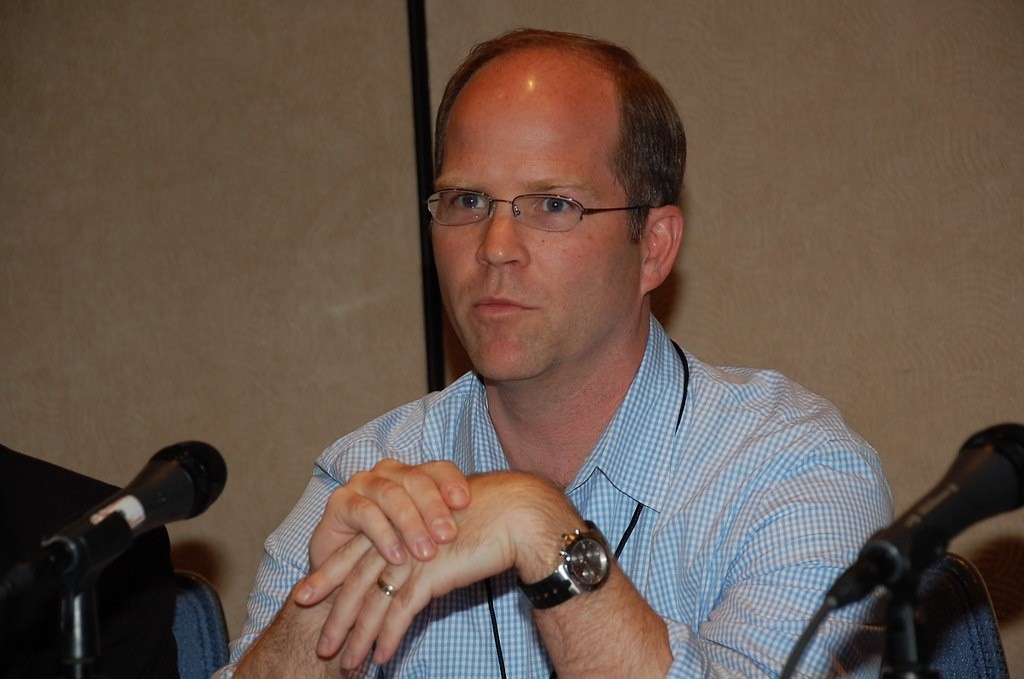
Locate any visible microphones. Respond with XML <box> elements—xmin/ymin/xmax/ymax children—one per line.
<box><xmin>826</xmin><ymin>422</ymin><xmax>1024</xmax><ymax>610</ymax></box>
<box><xmin>0</xmin><ymin>441</ymin><xmax>228</xmax><ymax>603</ymax></box>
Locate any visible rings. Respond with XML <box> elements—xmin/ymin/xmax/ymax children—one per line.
<box><xmin>377</xmin><ymin>578</ymin><xmax>393</xmax><ymax>596</ymax></box>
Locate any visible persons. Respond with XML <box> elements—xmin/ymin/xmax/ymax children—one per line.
<box><xmin>211</xmin><ymin>30</ymin><xmax>894</xmax><ymax>678</ymax></box>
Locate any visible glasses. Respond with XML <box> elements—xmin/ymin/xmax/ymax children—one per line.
<box><xmin>423</xmin><ymin>187</ymin><xmax>652</xmax><ymax>233</ymax></box>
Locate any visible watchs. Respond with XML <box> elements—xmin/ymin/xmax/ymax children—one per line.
<box><xmin>516</xmin><ymin>519</ymin><xmax>611</xmax><ymax>610</ymax></box>
